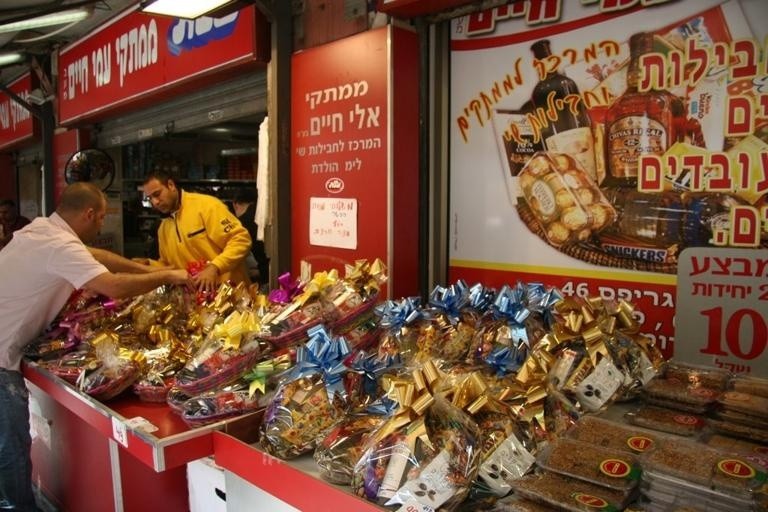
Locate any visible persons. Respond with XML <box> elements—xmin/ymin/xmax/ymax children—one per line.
<box><xmin>0</xmin><ymin>182</ymin><xmax>197</xmax><ymax>512</ymax></box>
<box><xmin>231</xmin><ymin>187</ymin><xmax>270</xmax><ymax>282</ymax></box>
<box><xmin>0</xmin><ymin>199</ymin><xmax>32</xmax><ymax>250</ymax></box>
<box><xmin>131</xmin><ymin>168</ymin><xmax>253</xmax><ymax>295</ymax></box>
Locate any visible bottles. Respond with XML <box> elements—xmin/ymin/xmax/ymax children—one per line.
<box><xmin>604</xmin><ymin>31</ymin><xmax>678</xmax><ymax>186</ymax></box>
<box><xmin>529</xmin><ymin>39</ymin><xmax>597</xmax><ymax>186</ymax></box>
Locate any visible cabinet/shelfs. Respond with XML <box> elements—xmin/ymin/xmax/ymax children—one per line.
<box><xmin>23</xmin><ymin>361</ymin><xmax>269</xmax><ymax>512</ymax></box>
<box><xmin>184</xmin><ymin>431</ymin><xmax>389</xmax><ymax>512</ymax></box>
